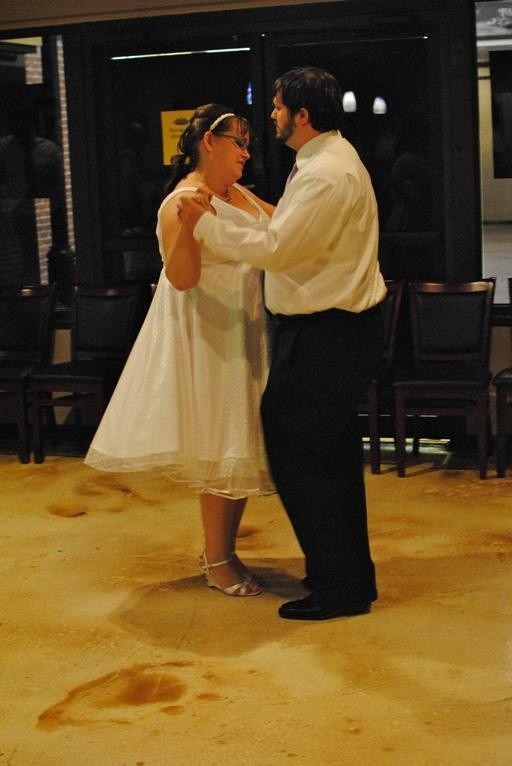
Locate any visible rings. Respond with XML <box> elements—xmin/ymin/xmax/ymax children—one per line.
<box><xmin>178</xmin><ymin>209</ymin><xmax>181</xmax><ymax>217</ymax></box>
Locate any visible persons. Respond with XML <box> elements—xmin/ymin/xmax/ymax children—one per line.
<box><xmin>83</xmin><ymin>103</ymin><xmax>276</xmax><ymax>596</ymax></box>
<box><xmin>177</xmin><ymin>67</ymin><xmax>388</xmax><ymax>620</ymax></box>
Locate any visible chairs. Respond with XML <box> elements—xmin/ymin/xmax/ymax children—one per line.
<box><xmin>0</xmin><ymin>281</ymin><xmax>144</xmax><ymax>464</ymax></box>
<box><xmin>356</xmin><ymin>278</ymin><xmax>511</xmax><ymax>480</ymax></box>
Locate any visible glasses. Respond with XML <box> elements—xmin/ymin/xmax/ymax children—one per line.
<box><xmin>214</xmin><ymin>133</ymin><xmax>249</xmax><ymax>147</ymax></box>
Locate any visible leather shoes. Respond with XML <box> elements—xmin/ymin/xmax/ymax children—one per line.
<box><xmin>279</xmin><ymin>577</ymin><xmax>378</xmax><ymax>620</ymax></box>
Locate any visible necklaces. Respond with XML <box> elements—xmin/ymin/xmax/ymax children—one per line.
<box><xmin>213</xmin><ymin>190</ymin><xmax>232</xmax><ymax>203</ymax></box>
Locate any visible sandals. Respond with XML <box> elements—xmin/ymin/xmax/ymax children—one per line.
<box><xmin>201</xmin><ymin>551</ymin><xmax>263</xmax><ymax>596</ymax></box>
